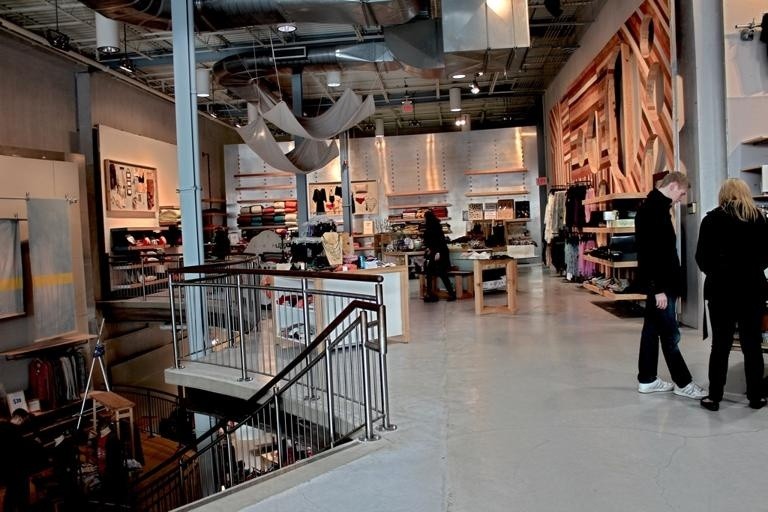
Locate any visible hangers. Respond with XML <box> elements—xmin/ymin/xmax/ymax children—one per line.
<box><xmin>564</xmin><ymin>232</ymin><xmax>597</xmax><ymax>248</ymax></box>
<box><xmin>550</xmin><ymin>181</ymin><xmax>595</xmax><ymax>198</ymax></box>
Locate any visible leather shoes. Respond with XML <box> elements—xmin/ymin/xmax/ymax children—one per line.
<box><xmin>700</xmin><ymin>396</ymin><xmax>719</xmax><ymax>410</ymax></box>
<box><xmin>748</xmin><ymin>397</ymin><xmax>767</xmax><ymax>409</ymax></box>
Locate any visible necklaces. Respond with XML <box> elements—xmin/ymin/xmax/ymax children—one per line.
<box><xmin>321</xmin><ymin>234</ymin><xmax>342</xmax><ymax>264</ymax></box>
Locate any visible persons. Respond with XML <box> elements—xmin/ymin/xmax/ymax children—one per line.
<box><xmin>0</xmin><ymin>408</ymin><xmax>42</xmax><ymax>511</ymax></box>
<box><xmin>420</xmin><ymin>211</ymin><xmax>455</xmax><ymax>301</ymax></box>
<box><xmin>51</xmin><ymin>435</ymin><xmax>81</xmax><ymax>506</ymax></box>
<box><xmin>210</xmin><ymin>226</ymin><xmax>230</xmax><ymax>259</ymax></box>
<box><xmin>95</xmin><ymin>425</ymin><xmax>129</xmax><ymax>505</ymax></box>
<box><xmin>632</xmin><ymin>168</ymin><xmax>708</xmax><ymax>399</ymax></box>
<box><xmin>691</xmin><ymin>177</ymin><xmax>768</xmax><ymax>411</ymax></box>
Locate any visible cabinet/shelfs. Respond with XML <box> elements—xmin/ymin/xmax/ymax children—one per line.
<box><xmin>107</xmin><ymin>224</ymin><xmax>188</xmax><ymax>296</ymax></box>
<box><xmin>271</xmin><ymin>263</ymin><xmax>411</xmax><ymax>358</ymax></box>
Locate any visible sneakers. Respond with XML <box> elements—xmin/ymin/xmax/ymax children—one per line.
<box><xmin>447</xmin><ymin>292</ymin><xmax>455</xmax><ymax>301</ymax></box>
<box><xmin>638</xmin><ymin>378</ymin><xmax>673</xmax><ymax>393</ymax></box>
<box><xmin>672</xmin><ymin>381</ymin><xmax>709</xmax><ymax>399</ymax></box>
<box><xmin>424</xmin><ymin>293</ymin><xmax>432</xmax><ymax>303</ymax></box>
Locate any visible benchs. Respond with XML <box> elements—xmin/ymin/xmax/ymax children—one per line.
<box><xmin>417</xmin><ymin>269</ymin><xmax>474</xmax><ymax>300</ymax></box>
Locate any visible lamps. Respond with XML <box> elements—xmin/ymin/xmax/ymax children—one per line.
<box><xmin>471</xmin><ymin>72</ymin><xmax>481</xmax><ymax>94</ymax></box>
<box><xmin>94</xmin><ymin>11</ymin><xmax>121</xmax><ymax>55</ymax></box>
<box><xmin>448</xmin><ymin>84</ymin><xmax>462</xmax><ymax>112</ymax></box>
<box><xmin>375</xmin><ymin>118</ymin><xmax>385</xmax><ymax>138</ymax></box>
